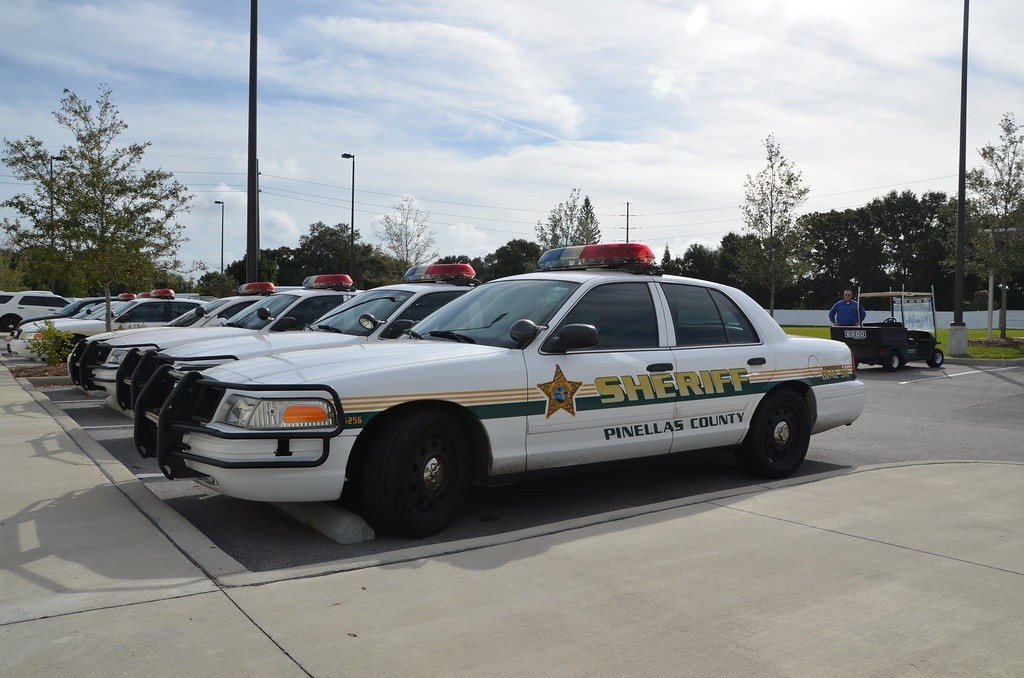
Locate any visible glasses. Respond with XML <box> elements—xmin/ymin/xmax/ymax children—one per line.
<box><xmin>843</xmin><ymin>293</ymin><xmax>852</xmax><ymax>296</ymax></box>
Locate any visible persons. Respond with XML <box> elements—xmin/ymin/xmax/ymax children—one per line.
<box><xmin>829</xmin><ymin>289</ymin><xmax>866</xmax><ymax>326</ymax></box>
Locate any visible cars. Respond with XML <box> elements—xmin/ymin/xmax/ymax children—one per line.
<box><xmin>136</xmin><ymin>243</ymin><xmax>868</xmax><ymax>540</ymax></box>
<box><xmin>0</xmin><ymin>265</ymin><xmax>548</xmax><ymax>459</ymax></box>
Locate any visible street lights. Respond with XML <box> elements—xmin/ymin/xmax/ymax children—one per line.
<box><xmin>341</xmin><ymin>152</ymin><xmax>356</xmax><ymax>275</ymax></box>
<box><xmin>215</xmin><ymin>200</ymin><xmax>225</xmax><ymax>274</ymax></box>
<box><xmin>49</xmin><ymin>153</ymin><xmax>70</xmax><ymax>248</ymax></box>
